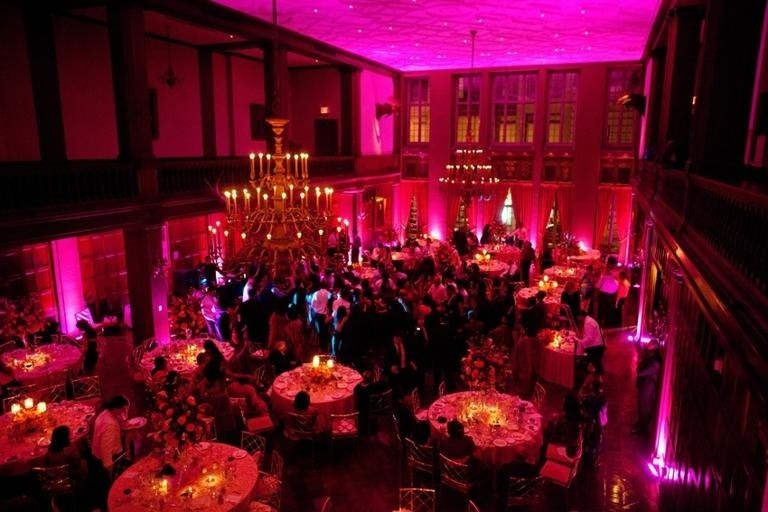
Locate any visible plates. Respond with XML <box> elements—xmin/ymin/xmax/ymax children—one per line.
<box><xmin>427</xmin><ymin>387</ymin><xmax>545</xmax><ymax>451</ymax></box>
<box><xmin>270</xmin><ymin>355</ymin><xmax>364</xmax><ymax>406</ymax></box>
<box><xmin>104</xmin><ymin>441</ymin><xmax>253</xmax><ymax>512</ymax></box>
<box><xmin>1</xmin><ymin>399</ymin><xmax>96</xmax><ymax>452</ymax></box>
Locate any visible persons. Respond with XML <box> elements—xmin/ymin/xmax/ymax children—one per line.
<box><xmin>1</xmin><ymin>223</ymin><xmax>667</xmax><ymax>511</ymax></box>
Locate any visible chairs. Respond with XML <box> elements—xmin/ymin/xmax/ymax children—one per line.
<box><xmin>4</xmin><ymin>241</ymin><xmax>607</xmax><ymax>512</ymax></box>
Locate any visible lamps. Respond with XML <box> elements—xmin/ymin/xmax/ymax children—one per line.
<box><xmin>436</xmin><ymin>31</ymin><xmax>501</xmax><ymax>204</ymax></box>
<box><xmin>205</xmin><ymin>0</ymin><xmax>350</xmax><ymax>279</ymax></box>
